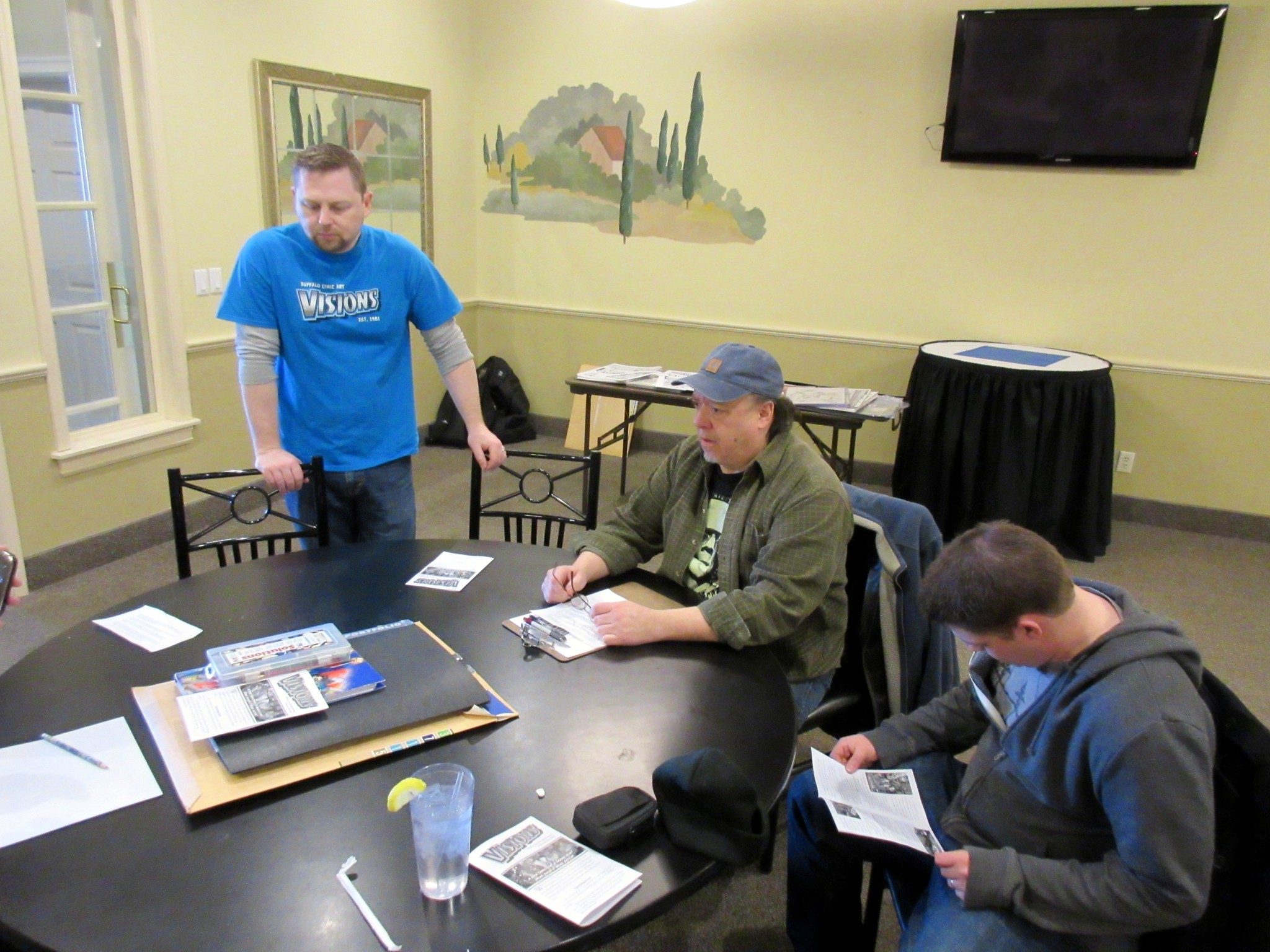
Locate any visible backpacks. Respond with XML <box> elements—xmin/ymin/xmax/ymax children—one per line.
<box><xmin>425</xmin><ymin>356</ymin><xmax>537</xmax><ymax>448</ymax></box>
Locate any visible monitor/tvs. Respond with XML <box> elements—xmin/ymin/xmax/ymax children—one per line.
<box><xmin>942</xmin><ymin>5</ymin><xmax>1229</xmax><ymax>169</ymax></box>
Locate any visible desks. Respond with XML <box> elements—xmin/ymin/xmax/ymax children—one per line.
<box><xmin>892</xmin><ymin>340</ymin><xmax>1116</xmax><ymax>564</ymax></box>
<box><xmin>0</xmin><ymin>539</ymin><xmax>795</xmax><ymax>951</ymax></box>
<box><xmin>568</xmin><ymin>375</ymin><xmax>904</xmax><ymax>512</ymax></box>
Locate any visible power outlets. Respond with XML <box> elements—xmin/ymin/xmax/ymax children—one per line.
<box><xmin>1115</xmin><ymin>451</ymin><xmax>1135</xmax><ymax>473</ymax></box>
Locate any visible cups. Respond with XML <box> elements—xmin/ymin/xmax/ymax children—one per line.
<box><xmin>408</xmin><ymin>762</ymin><xmax>474</xmax><ymax>901</ymax></box>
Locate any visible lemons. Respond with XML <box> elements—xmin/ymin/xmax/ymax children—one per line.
<box><xmin>387</xmin><ymin>777</ymin><xmax>427</xmax><ymax>812</ymax></box>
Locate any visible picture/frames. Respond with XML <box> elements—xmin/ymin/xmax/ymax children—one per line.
<box><xmin>255</xmin><ymin>59</ymin><xmax>435</xmax><ymax>267</ymax></box>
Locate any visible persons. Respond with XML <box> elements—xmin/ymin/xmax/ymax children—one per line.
<box><xmin>538</xmin><ymin>343</ymin><xmax>853</xmax><ymax>731</ymax></box>
<box><xmin>786</xmin><ymin>518</ymin><xmax>1217</xmax><ymax>951</ymax></box>
<box><xmin>213</xmin><ymin>143</ymin><xmax>507</xmax><ymax>552</ymax></box>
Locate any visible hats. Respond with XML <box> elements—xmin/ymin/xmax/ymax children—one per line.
<box><xmin>670</xmin><ymin>342</ymin><xmax>784</xmax><ymax>404</ymax></box>
<box><xmin>652</xmin><ymin>747</ymin><xmax>769</xmax><ymax>868</ymax></box>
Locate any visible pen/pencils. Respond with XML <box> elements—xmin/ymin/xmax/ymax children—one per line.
<box><xmin>523</xmin><ymin>614</ymin><xmax>567</xmax><ymax>642</ymax></box>
<box><xmin>40</xmin><ymin>733</ymin><xmax>108</xmax><ymax>770</ymax></box>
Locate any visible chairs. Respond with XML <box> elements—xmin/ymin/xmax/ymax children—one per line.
<box><xmin>759</xmin><ymin>483</ymin><xmax>959</xmax><ymax>873</ymax></box>
<box><xmin>468</xmin><ymin>451</ymin><xmax>601</xmax><ymax>547</ymax></box>
<box><xmin>865</xmin><ymin>665</ymin><xmax>1270</xmax><ymax>952</ymax></box>
<box><xmin>167</xmin><ymin>454</ymin><xmax>329</xmax><ymax>582</ymax></box>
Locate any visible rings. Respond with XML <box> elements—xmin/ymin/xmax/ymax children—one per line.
<box><xmin>951</xmin><ymin>878</ymin><xmax>954</xmax><ymax>888</ymax></box>
<box><xmin>501</xmin><ymin>448</ymin><xmax>506</xmax><ymax>452</ymax></box>
